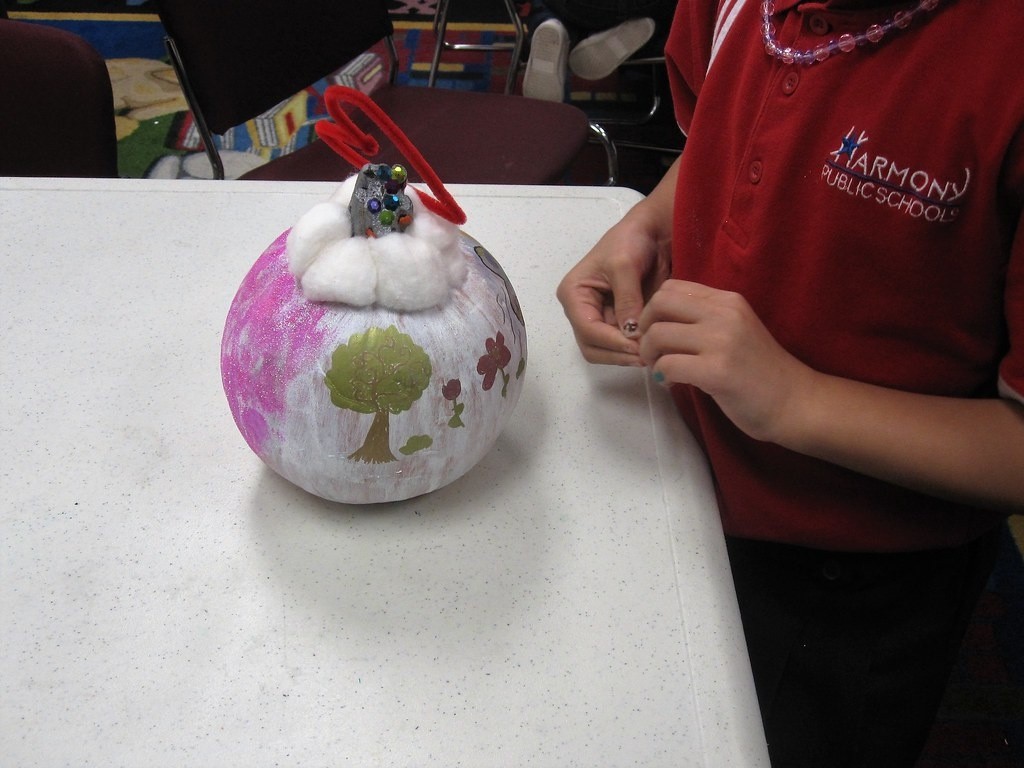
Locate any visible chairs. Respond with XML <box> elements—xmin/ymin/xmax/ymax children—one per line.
<box><xmin>0</xmin><ymin>0</ymin><xmax>668</xmax><ymax>189</ymax></box>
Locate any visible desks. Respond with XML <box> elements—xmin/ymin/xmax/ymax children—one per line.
<box><xmin>3</xmin><ymin>178</ymin><xmax>780</xmax><ymax>767</ymax></box>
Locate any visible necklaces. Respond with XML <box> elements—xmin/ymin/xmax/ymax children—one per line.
<box><xmin>758</xmin><ymin>0</ymin><xmax>937</xmax><ymax>65</ymax></box>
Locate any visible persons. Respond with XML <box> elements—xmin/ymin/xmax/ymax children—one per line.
<box><xmin>557</xmin><ymin>0</ymin><xmax>1024</xmax><ymax>768</ymax></box>
<box><xmin>520</xmin><ymin>0</ymin><xmax>677</xmax><ymax>105</ymax></box>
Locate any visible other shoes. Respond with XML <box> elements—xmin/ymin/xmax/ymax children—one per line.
<box><xmin>522</xmin><ymin>17</ymin><xmax>572</xmax><ymax>104</ymax></box>
<box><xmin>569</xmin><ymin>19</ymin><xmax>657</xmax><ymax>81</ymax></box>
